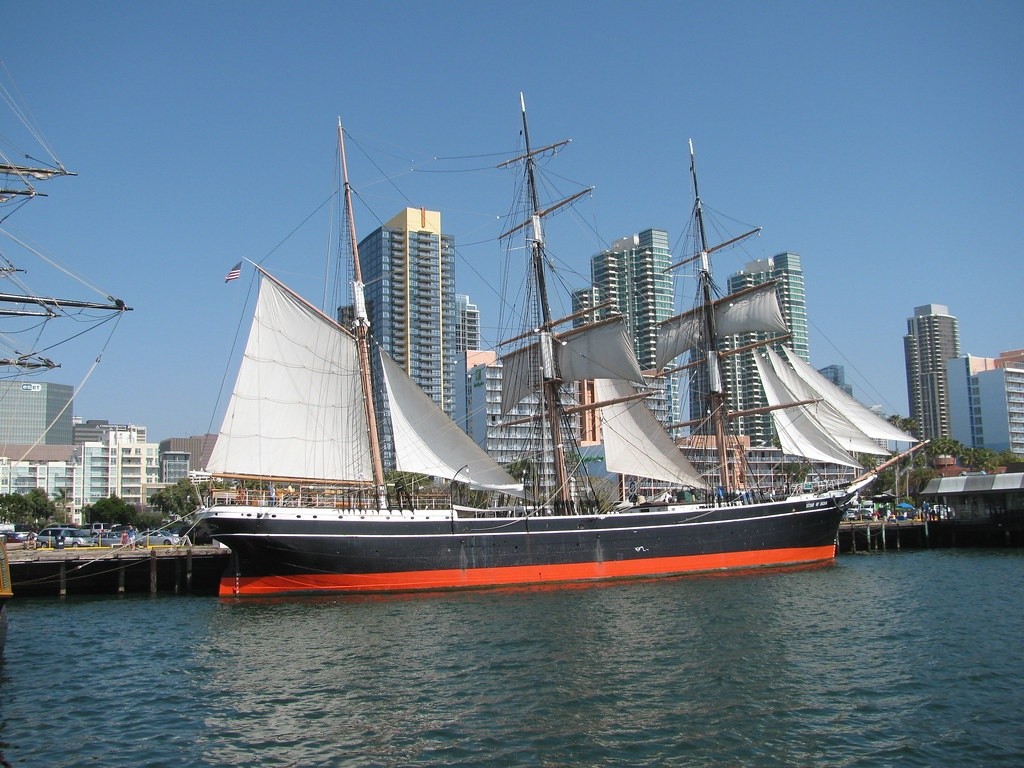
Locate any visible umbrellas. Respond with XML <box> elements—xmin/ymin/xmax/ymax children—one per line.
<box><xmin>896</xmin><ymin>503</ymin><xmax>915</xmax><ymax>509</ymax></box>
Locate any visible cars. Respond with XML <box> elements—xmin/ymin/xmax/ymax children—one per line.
<box><xmin>93</xmin><ymin>532</ymin><xmax>121</xmax><ymax>547</ymax></box>
<box><xmin>0</xmin><ymin>532</ymin><xmax>38</xmax><ymax>543</ymax></box>
<box><xmin>79</xmin><ymin>523</ymin><xmax>138</xmax><ymax>538</ymax></box>
<box><xmin>135</xmin><ymin>530</ymin><xmax>180</xmax><ymax>546</ymax></box>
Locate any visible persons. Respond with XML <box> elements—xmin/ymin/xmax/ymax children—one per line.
<box><xmin>287</xmin><ymin>483</ymin><xmax>292</xmax><ymax>500</ymax></box>
<box><xmin>129</xmin><ymin>526</ymin><xmax>134</xmax><ymax>550</ymax></box>
<box><xmin>26</xmin><ymin>532</ymin><xmax>37</xmax><ymax>550</ymax></box>
<box><xmin>121</xmin><ymin>531</ymin><xmax>128</xmax><ymax>547</ymax></box>
<box><xmin>270</xmin><ymin>485</ymin><xmax>275</xmax><ymax>506</ymax></box>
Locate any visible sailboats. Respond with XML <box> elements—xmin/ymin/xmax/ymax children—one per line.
<box><xmin>0</xmin><ymin>56</ymin><xmax>134</xmax><ymax>651</ymax></box>
<box><xmin>196</xmin><ymin>91</ymin><xmax>932</xmax><ymax>598</ymax></box>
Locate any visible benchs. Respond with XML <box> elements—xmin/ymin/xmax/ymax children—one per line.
<box><xmin>110</xmin><ymin>544</ymin><xmax>123</xmax><ymax>548</ymax></box>
<box><xmin>24</xmin><ymin>541</ymin><xmax>41</xmax><ymax>549</ymax></box>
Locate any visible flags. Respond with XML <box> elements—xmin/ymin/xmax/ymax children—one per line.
<box><xmin>225</xmin><ymin>261</ymin><xmax>243</xmax><ymax>283</ymax></box>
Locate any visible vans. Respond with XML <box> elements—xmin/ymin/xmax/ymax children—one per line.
<box><xmin>37</xmin><ymin>528</ymin><xmax>94</xmax><ymax>548</ymax></box>
